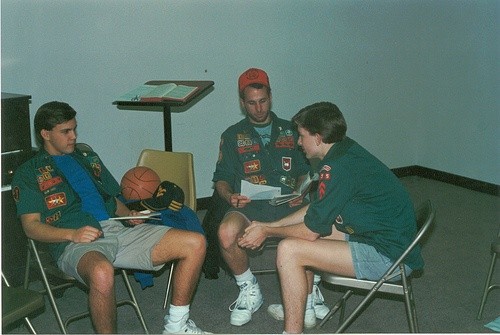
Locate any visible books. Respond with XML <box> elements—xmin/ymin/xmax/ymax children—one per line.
<box><xmin>142</xmin><ymin>83</ymin><xmax>199</xmax><ymax>103</ymax></box>
<box><xmin>269</xmin><ymin>179</ymin><xmax>313</xmax><ymax>206</ymax></box>
<box><xmin>109</xmin><ymin>213</ymin><xmax>162</xmax><ymax>221</ymax></box>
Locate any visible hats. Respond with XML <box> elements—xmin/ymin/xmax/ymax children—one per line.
<box><xmin>140</xmin><ymin>181</ymin><xmax>184</xmax><ymax>212</ymax></box>
<box><xmin>237</xmin><ymin>68</ymin><xmax>271</xmax><ymax>94</ymax></box>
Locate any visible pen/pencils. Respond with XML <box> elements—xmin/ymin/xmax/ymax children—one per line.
<box><xmin>237</xmin><ymin>194</ymin><xmax>240</xmax><ymax>207</ymax></box>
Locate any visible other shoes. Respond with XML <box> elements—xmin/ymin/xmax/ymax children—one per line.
<box><xmin>267</xmin><ymin>302</ymin><xmax>316</xmax><ymax>328</ymax></box>
<box><xmin>162</xmin><ymin>314</ymin><xmax>214</xmax><ymax>335</ymax></box>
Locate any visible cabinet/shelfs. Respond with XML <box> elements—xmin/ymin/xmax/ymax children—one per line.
<box><xmin>1</xmin><ymin>92</ymin><xmax>36</xmax><ymax>285</ymax></box>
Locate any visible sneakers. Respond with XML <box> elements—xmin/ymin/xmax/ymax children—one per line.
<box><xmin>228</xmin><ymin>280</ymin><xmax>263</xmax><ymax>327</ymax></box>
<box><xmin>311</xmin><ymin>284</ymin><xmax>333</xmax><ymax>321</ymax></box>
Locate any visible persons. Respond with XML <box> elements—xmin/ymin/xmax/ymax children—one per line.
<box><xmin>238</xmin><ymin>101</ymin><xmax>424</xmax><ymax>335</ymax></box>
<box><xmin>11</xmin><ymin>101</ymin><xmax>213</xmax><ymax>335</ymax></box>
<box><xmin>212</xmin><ymin>67</ymin><xmax>330</xmax><ymax>326</ymax></box>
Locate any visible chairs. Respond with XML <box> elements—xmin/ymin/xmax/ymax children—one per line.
<box><xmin>2</xmin><ymin>151</ymin><xmax>436</xmax><ymax>335</ymax></box>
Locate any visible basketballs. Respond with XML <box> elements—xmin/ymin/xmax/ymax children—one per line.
<box><xmin>119</xmin><ymin>165</ymin><xmax>163</xmax><ymax>204</ymax></box>
